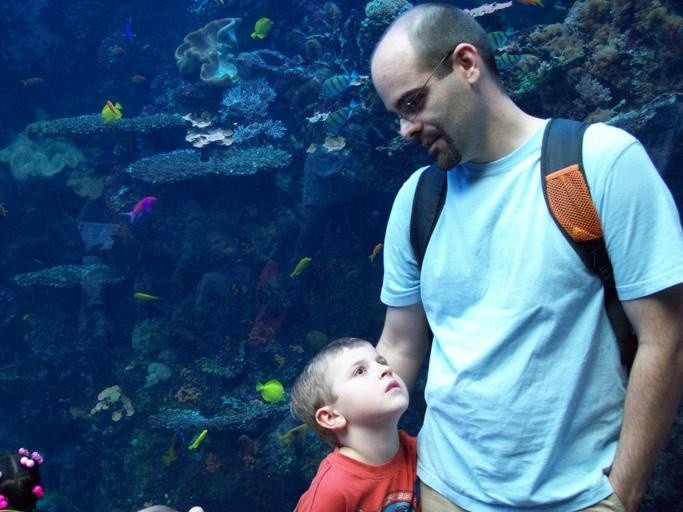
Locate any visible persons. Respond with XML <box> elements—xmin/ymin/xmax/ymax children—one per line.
<box><xmin>366</xmin><ymin>1</ymin><xmax>682</xmax><ymax>512</ymax></box>
<box><xmin>0</xmin><ymin>447</ymin><xmax>45</xmax><ymax>511</ymax></box>
<box><xmin>288</xmin><ymin>335</ymin><xmax>424</xmax><ymax>512</ymax></box>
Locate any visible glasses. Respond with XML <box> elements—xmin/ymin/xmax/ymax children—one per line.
<box><xmin>396</xmin><ymin>50</ymin><xmax>453</xmax><ymax>123</ymax></box>
